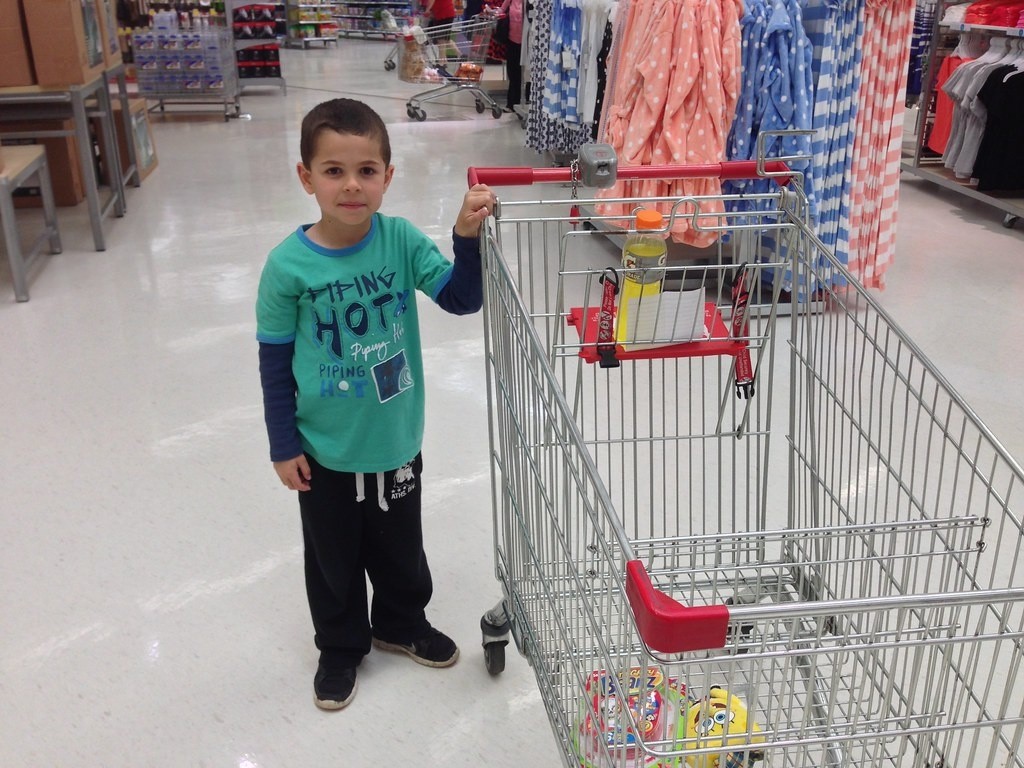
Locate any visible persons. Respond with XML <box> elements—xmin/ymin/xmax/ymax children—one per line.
<box><xmin>483</xmin><ymin>0</ymin><xmax>524</xmax><ymax>112</ymax></box>
<box><xmin>418</xmin><ymin>0</ymin><xmax>455</xmax><ymax>67</ymax></box>
<box><xmin>255</xmin><ymin>99</ymin><xmax>495</xmax><ymax>710</ymax></box>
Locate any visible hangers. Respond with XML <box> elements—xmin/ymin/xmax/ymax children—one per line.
<box><xmin>949</xmin><ymin>32</ymin><xmax>1024</xmax><ymax>82</ymax></box>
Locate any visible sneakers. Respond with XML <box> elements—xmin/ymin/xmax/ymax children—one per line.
<box><xmin>313</xmin><ymin>660</ymin><xmax>359</xmax><ymax>710</ymax></box>
<box><xmin>371</xmin><ymin>619</ymin><xmax>459</xmax><ymax>668</ymax></box>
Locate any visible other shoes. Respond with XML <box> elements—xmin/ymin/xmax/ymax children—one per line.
<box><xmin>433</xmin><ymin>63</ymin><xmax>448</xmax><ymax>69</ymax></box>
<box><xmin>459</xmin><ymin>54</ymin><xmax>468</xmax><ymax>62</ymax></box>
<box><xmin>504</xmin><ymin>106</ymin><xmax>515</xmax><ymax>113</ymax></box>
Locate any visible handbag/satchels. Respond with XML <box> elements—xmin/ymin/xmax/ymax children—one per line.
<box><xmin>494</xmin><ymin>0</ymin><xmax>511</xmax><ymax>44</ymax></box>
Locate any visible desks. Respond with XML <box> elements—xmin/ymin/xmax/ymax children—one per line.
<box><xmin>1</xmin><ymin>76</ymin><xmax>127</xmax><ymax>252</ymax></box>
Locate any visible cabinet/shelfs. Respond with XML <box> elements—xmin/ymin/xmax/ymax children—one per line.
<box><xmin>332</xmin><ymin>2</ymin><xmax>409</xmax><ymax>36</ymax></box>
<box><xmin>225</xmin><ymin>0</ymin><xmax>287</xmax><ymax>96</ymax></box>
<box><xmin>288</xmin><ymin>4</ymin><xmax>339</xmax><ymax>49</ymax></box>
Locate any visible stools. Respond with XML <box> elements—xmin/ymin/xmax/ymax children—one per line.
<box><xmin>0</xmin><ymin>143</ymin><xmax>63</xmax><ymax>302</ymax></box>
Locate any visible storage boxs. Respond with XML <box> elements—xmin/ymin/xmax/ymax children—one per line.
<box><xmin>23</xmin><ymin>0</ymin><xmax>106</xmax><ymax>88</ymax></box>
<box><xmin>0</xmin><ymin>0</ymin><xmax>33</xmax><ymax>87</ymax></box>
<box><xmin>85</xmin><ymin>100</ymin><xmax>158</xmax><ymax>186</ymax></box>
<box><xmin>96</xmin><ymin>0</ymin><xmax>123</xmax><ymax>68</ymax></box>
<box><xmin>0</xmin><ymin>121</ymin><xmax>83</xmax><ymax>206</ymax></box>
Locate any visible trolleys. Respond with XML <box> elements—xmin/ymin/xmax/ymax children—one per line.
<box><xmin>130</xmin><ymin>29</ymin><xmax>242</xmax><ymax>124</ymax></box>
<box><xmin>467</xmin><ymin>142</ymin><xmax>1024</xmax><ymax>768</ymax></box>
<box><xmin>394</xmin><ymin>6</ymin><xmax>505</xmax><ymax>122</ymax></box>
<box><xmin>383</xmin><ymin>11</ymin><xmax>440</xmax><ymax>71</ymax></box>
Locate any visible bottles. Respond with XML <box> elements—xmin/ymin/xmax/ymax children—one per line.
<box><xmin>131</xmin><ymin>8</ymin><xmax>239</xmax><ymax>94</ymax></box>
<box><xmin>612</xmin><ymin>209</ymin><xmax>668</xmax><ymax>353</ymax></box>
